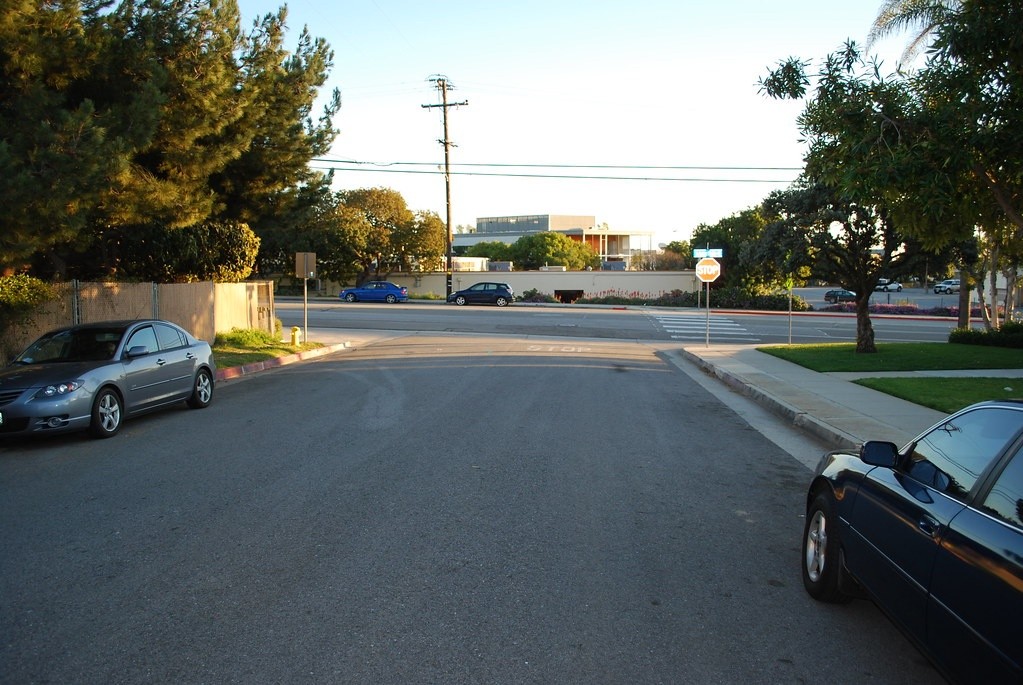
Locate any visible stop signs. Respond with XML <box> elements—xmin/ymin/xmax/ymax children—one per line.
<box><xmin>696</xmin><ymin>258</ymin><xmax>721</xmax><ymax>282</ymax></box>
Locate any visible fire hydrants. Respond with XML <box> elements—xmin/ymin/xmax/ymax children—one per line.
<box><xmin>290</xmin><ymin>326</ymin><xmax>303</xmax><ymax>348</ymax></box>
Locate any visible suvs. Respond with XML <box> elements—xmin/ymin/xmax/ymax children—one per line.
<box><xmin>824</xmin><ymin>288</ymin><xmax>858</xmax><ymax>304</ymax></box>
<box><xmin>933</xmin><ymin>279</ymin><xmax>961</xmax><ymax>296</ymax></box>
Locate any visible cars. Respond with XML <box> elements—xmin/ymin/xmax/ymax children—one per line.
<box><xmin>801</xmin><ymin>398</ymin><xmax>1023</xmax><ymax>685</ymax></box>
<box><xmin>875</xmin><ymin>278</ymin><xmax>904</xmax><ymax>292</ymax></box>
<box><xmin>447</xmin><ymin>282</ymin><xmax>515</xmax><ymax>306</ymax></box>
<box><xmin>0</xmin><ymin>319</ymin><xmax>219</xmax><ymax>440</ymax></box>
<box><xmin>339</xmin><ymin>280</ymin><xmax>409</xmax><ymax>304</ymax></box>
